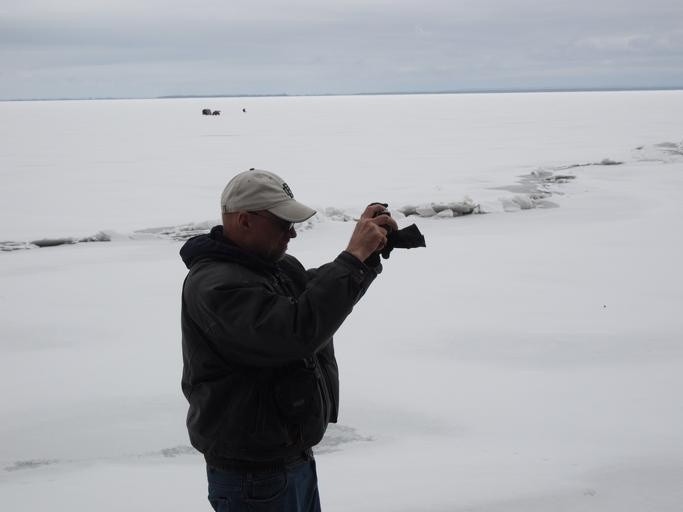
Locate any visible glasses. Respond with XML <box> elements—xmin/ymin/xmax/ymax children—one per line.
<box><xmin>250</xmin><ymin>210</ymin><xmax>294</xmax><ymax>231</ymax></box>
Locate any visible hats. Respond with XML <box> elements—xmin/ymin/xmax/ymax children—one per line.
<box><xmin>221</xmin><ymin>168</ymin><xmax>316</xmax><ymax>222</ymax></box>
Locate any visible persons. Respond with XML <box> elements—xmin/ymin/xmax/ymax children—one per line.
<box><xmin>179</xmin><ymin>167</ymin><xmax>399</xmax><ymax>512</ymax></box>
